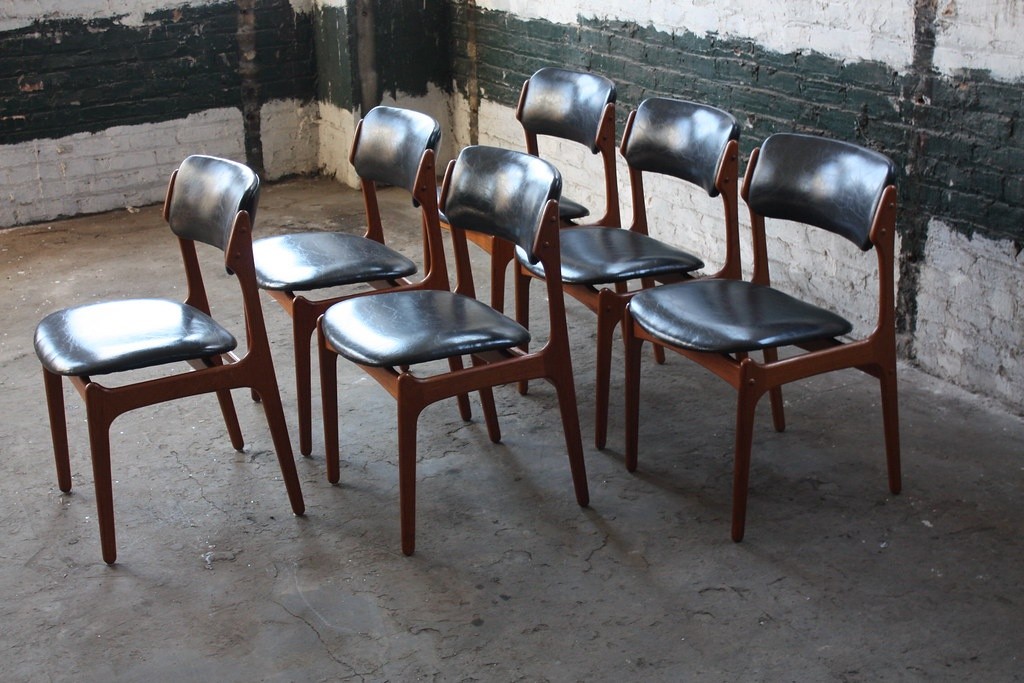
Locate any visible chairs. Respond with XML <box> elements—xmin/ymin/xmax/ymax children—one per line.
<box><xmin>316</xmin><ymin>144</ymin><xmax>590</xmax><ymax>557</ymax></box>
<box><xmin>245</xmin><ymin>106</ymin><xmax>472</xmax><ymax>455</ymax></box>
<box><xmin>422</xmin><ymin>66</ymin><xmax>622</xmax><ymax>316</ymax></box>
<box><xmin>625</xmin><ymin>133</ymin><xmax>902</xmax><ymax>541</ymax></box>
<box><xmin>512</xmin><ymin>95</ymin><xmax>750</xmax><ymax>452</ymax></box>
<box><xmin>34</xmin><ymin>154</ymin><xmax>305</xmax><ymax>562</ymax></box>
